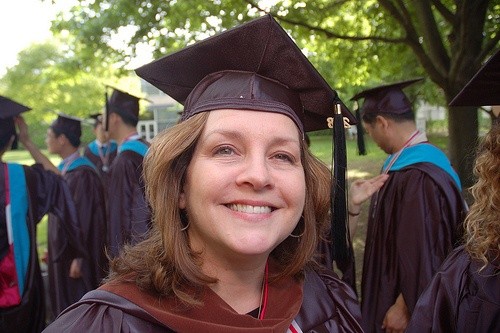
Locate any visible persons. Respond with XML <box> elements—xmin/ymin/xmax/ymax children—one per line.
<box><xmin>408</xmin><ymin>134</ymin><xmax>496</xmax><ymax>332</ymax></box>
<box><xmin>44</xmin><ymin>115</ymin><xmax>103</xmax><ymax>332</ymax></box>
<box><xmin>1</xmin><ymin>96</ymin><xmax>61</xmax><ymax>332</ymax></box>
<box><xmin>351</xmin><ymin>78</ymin><xmax>469</xmax><ymax>333</ymax></box>
<box><xmin>105</xmin><ymin>86</ymin><xmax>152</xmax><ymax>261</ymax></box>
<box><xmin>88</xmin><ymin>110</ymin><xmax>387</xmax><ymax>245</ymax></box>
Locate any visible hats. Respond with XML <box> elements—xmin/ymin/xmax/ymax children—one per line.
<box><xmin>88</xmin><ymin>112</ymin><xmax>105</xmax><ymax>126</ymax></box>
<box><xmin>134</xmin><ymin>14</ymin><xmax>359</xmax><ymax>262</ymax></box>
<box><xmin>349</xmin><ymin>77</ymin><xmax>426</xmax><ymax>157</ymax></box>
<box><xmin>447</xmin><ymin>47</ymin><xmax>500</xmax><ymax>109</ymax></box>
<box><xmin>48</xmin><ymin>111</ymin><xmax>83</xmax><ymax>137</ymax></box>
<box><xmin>99</xmin><ymin>79</ymin><xmax>153</xmax><ymax>132</ymax></box>
<box><xmin>0</xmin><ymin>96</ymin><xmax>32</xmax><ymax>150</ymax></box>
